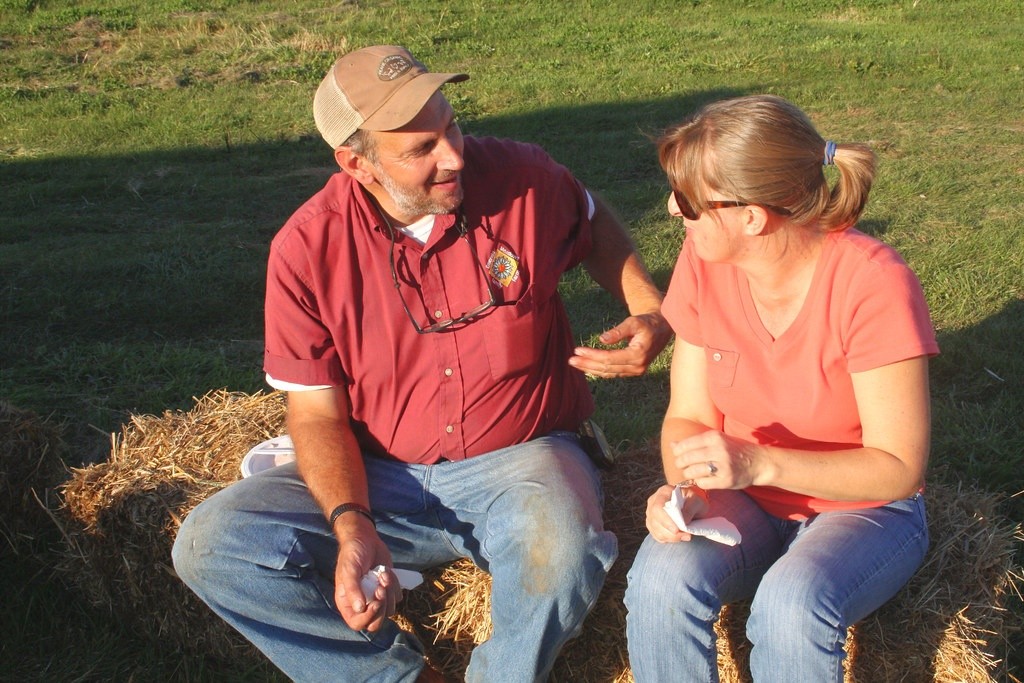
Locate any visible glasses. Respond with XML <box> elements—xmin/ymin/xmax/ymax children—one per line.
<box><xmin>674</xmin><ymin>189</ymin><xmax>791</xmax><ymax>220</ymax></box>
<box><xmin>390</xmin><ymin>232</ymin><xmax>496</xmax><ymax>334</ymax></box>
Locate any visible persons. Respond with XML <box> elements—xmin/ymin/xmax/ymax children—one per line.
<box><xmin>622</xmin><ymin>92</ymin><xmax>941</xmax><ymax>682</ymax></box>
<box><xmin>170</xmin><ymin>44</ymin><xmax>673</xmax><ymax>683</ymax></box>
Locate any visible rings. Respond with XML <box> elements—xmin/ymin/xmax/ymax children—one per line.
<box><xmin>614</xmin><ymin>373</ymin><xmax>619</xmax><ymax>379</ymax></box>
<box><xmin>708</xmin><ymin>464</ymin><xmax>716</xmax><ymax>477</ymax></box>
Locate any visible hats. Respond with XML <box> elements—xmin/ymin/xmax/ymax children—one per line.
<box><xmin>313</xmin><ymin>45</ymin><xmax>471</xmax><ymax>150</ymax></box>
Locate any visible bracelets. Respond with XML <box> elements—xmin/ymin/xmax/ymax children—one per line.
<box><xmin>328</xmin><ymin>502</ymin><xmax>376</xmax><ymax>532</ymax></box>
